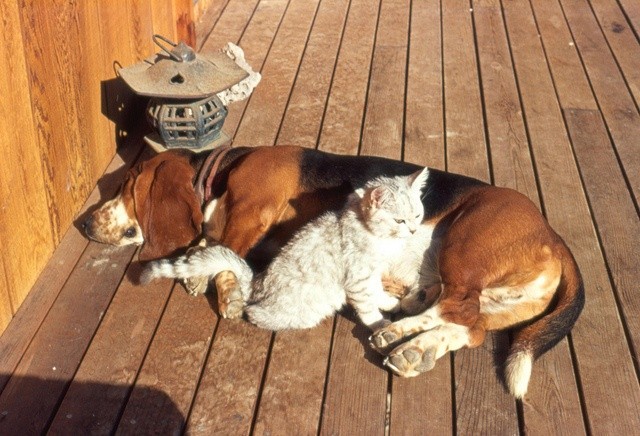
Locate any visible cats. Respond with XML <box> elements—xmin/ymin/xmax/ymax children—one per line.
<box><xmin>137</xmin><ymin>164</ymin><xmax>435</xmax><ymax>332</ymax></box>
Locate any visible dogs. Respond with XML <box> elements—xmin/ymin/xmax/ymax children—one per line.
<box><xmin>79</xmin><ymin>143</ymin><xmax>586</xmax><ymax>400</ymax></box>
